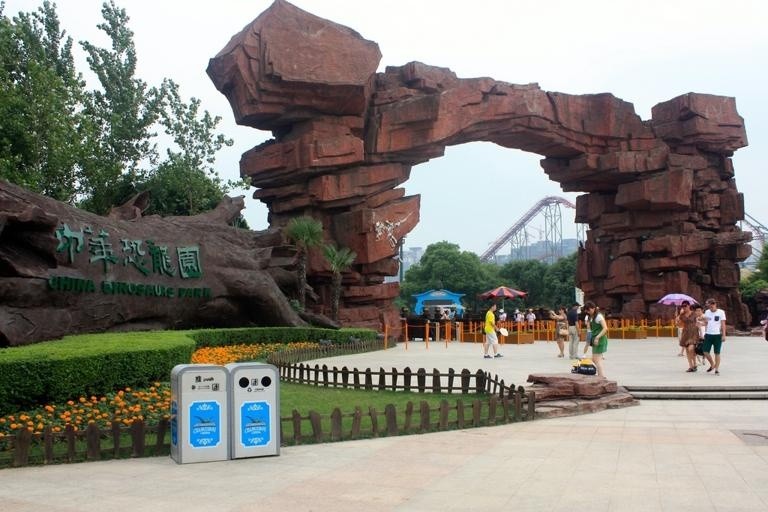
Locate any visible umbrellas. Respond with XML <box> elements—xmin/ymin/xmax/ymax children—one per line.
<box><xmin>483</xmin><ymin>287</ymin><xmax>528</xmax><ymax>311</ymax></box>
<box><xmin>658</xmin><ymin>294</ymin><xmax>698</xmax><ymax>321</ymax></box>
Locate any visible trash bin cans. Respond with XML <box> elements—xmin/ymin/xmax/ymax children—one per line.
<box><xmin>170</xmin><ymin>362</ymin><xmax>281</xmax><ymax>464</ymax></box>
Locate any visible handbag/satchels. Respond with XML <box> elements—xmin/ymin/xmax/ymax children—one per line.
<box><xmin>696</xmin><ymin>338</ymin><xmax>705</xmax><ymax>356</ymax></box>
<box><xmin>559</xmin><ymin>329</ymin><xmax>568</xmax><ymax>336</ymax></box>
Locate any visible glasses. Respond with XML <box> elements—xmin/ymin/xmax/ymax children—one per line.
<box><xmin>681</xmin><ymin>304</ymin><xmax>687</xmax><ymax>308</ymax></box>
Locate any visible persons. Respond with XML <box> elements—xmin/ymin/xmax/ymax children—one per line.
<box><xmin>549</xmin><ymin>301</ymin><xmax>594</xmax><ymax>359</ymax></box>
<box><xmin>420</xmin><ymin>305</ymin><xmax>461</xmax><ymax>323</ymax></box>
<box><xmin>585</xmin><ymin>305</ymin><xmax>610</xmax><ymax>378</ymax></box>
<box><xmin>498</xmin><ymin>309</ymin><xmax>538</xmax><ymax>330</ymax></box>
<box><xmin>484</xmin><ymin>303</ymin><xmax>506</xmax><ymax>360</ymax></box>
<box><xmin>678</xmin><ymin>300</ymin><xmax>726</xmax><ymax>375</ymax></box>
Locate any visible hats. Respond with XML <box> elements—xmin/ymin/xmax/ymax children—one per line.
<box><xmin>529</xmin><ymin>309</ymin><xmax>533</xmax><ymax>312</ymax></box>
<box><xmin>573</xmin><ymin>302</ymin><xmax>581</xmax><ymax>306</ymax></box>
<box><xmin>514</xmin><ymin>309</ymin><xmax>520</xmax><ymax>315</ymax></box>
<box><xmin>704</xmin><ymin>298</ymin><xmax>719</xmax><ymax>308</ymax></box>
<box><xmin>499</xmin><ymin>309</ymin><xmax>504</xmax><ymax>313</ymax></box>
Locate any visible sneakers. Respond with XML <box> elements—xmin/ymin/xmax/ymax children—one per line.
<box><xmin>494</xmin><ymin>354</ymin><xmax>502</xmax><ymax>357</ymax></box>
<box><xmin>687</xmin><ymin>367</ymin><xmax>696</xmax><ymax>372</ymax></box>
<box><xmin>484</xmin><ymin>355</ymin><xmax>493</xmax><ymax>358</ymax></box>
<box><xmin>715</xmin><ymin>372</ymin><xmax>719</xmax><ymax>373</ymax></box>
<box><xmin>707</xmin><ymin>365</ymin><xmax>715</xmax><ymax>372</ymax></box>
